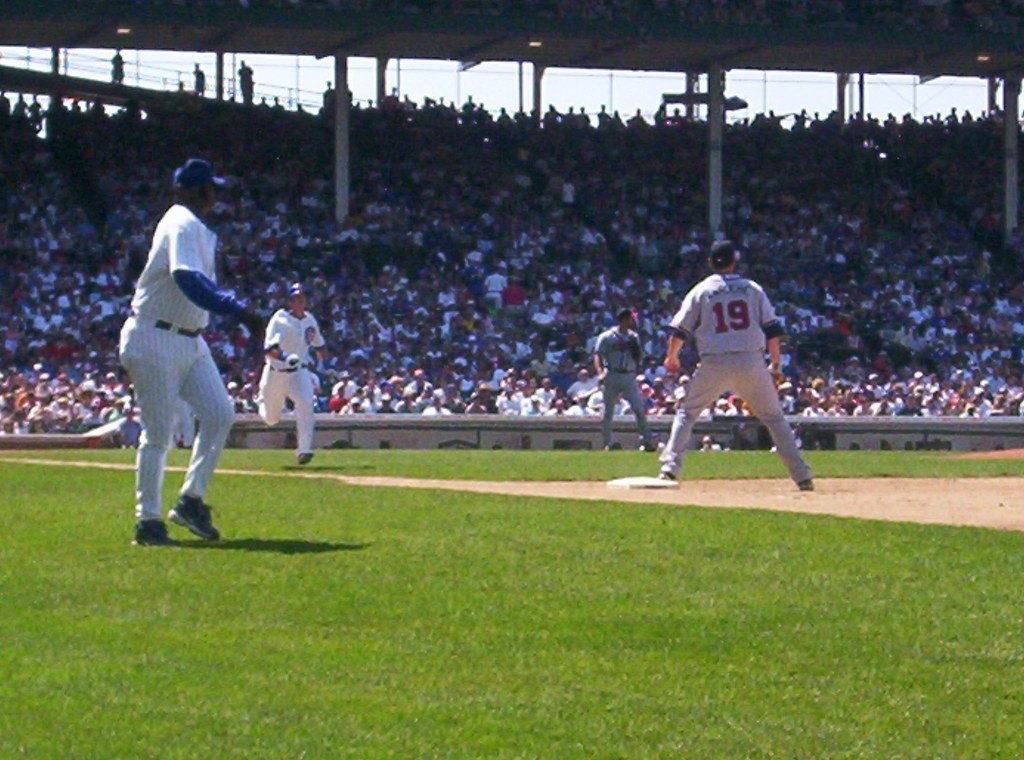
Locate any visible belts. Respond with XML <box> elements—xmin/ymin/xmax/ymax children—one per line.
<box><xmin>131</xmin><ymin>310</ymin><xmax>202</xmax><ymax>338</ymax></box>
<box><xmin>271</xmin><ymin>366</ymin><xmax>298</xmax><ymax>372</ymax></box>
<box><xmin>608</xmin><ymin>368</ymin><xmax>636</xmax><ymax>374</ymax></box>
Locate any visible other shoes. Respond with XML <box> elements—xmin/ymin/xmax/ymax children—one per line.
<box><xmin>799</xmin><ymin>479</ymin><xmax>813</xmax><ymax>490</ymax></box>
<box><xmin>298</xmin><ymin>453</ymin><xmax>312</xmax><ymax>464</ymax></box>
<box><xmin>660</xmin><ymin>472</ymin><xmax>675</xmax><ymax>480</ymax></box>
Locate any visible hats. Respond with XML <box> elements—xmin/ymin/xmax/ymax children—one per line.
<box><xmin>174</xmin><ymin>157</ymin><xmax>226</xmax><ymax>187</ymax></box>
<box><xmin>288</xmin><ymin>283</ymin><xmax>306</xmax><ymax>295</ymax></box>
<box><xmin>617</xmin><ymin>309</ymin><xmax>638</xmax><ymax>320</ymax></box>
<box><xmin>710</xmin><ymin>240</ymin><xmax>739</xmax><ymax>264</ymax></box>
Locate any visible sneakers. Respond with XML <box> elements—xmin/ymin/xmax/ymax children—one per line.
<box><xmin>136</xmin><ymin>520</ymin><xmax>167</xmax><ymax>547</ymax></box>
<box><xmin>168</xmin><ymin>495</ymin><xmax>218</xmax><ymax>539</ymax></box>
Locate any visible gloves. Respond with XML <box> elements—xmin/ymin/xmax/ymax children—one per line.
<box><xmin>327</xmin><ymin>368</ymin><xmax>339</xmax><ymax>384</ymax></box>
<box><xmin>286</xmin><ymin>354</ymin><xmax>299</xmax><ymax>369</ymax></box>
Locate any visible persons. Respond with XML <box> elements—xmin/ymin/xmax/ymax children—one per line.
<box><xmin>193</xmin><ymin>63</ymin><xmax>206</xmax><ymax>97</ymax></box>
<box><xmin>593</xmin><ymin>309</ymin><xmax>657</xmax><ymax>452</ymax></box>
<box><xmin>0</xmin><ymin>91</ymin><xmax>186</xmax><ymax>434</ymax></box>
<box><xmin>257</xmin><ymin>282</ymin><xmax>331</xmax><ymax>463</ymax></box>
<box><xmin>237</xmin><ymin>60</ymin><xmax>254</xmax><ymax>105</ymax></box>
<box><xmin>839</xmin><ymin>106</ymin><xmax>1023</xmax><ymax>417</ymax></box>
<box><xmin>637</xmin><ymin>422</ymin><xmax>821</xmax><ymax>452</ymax></box>
<box><xmin>183</xmin><ymin>106</ymin><xmax>364</xmax><ymax>414</ymax></box>
<box><xmin>323</xmin><ymin>82</ymin><xmax>605</xmax><ymax>416</ymax></box>
<box><xmin>117</xmin><ymin>160</ymin><xmax>262</xmax><ymax>548</ymax></box>
<box><xmin>119</xmin><ymin>412</ymin><xmax>142</xmax><ymax>449</ymax></box>
<box><xmin>597</xmin><ymin>102</ymin><xmax>852</xmax><ymax>415</ymax></box>
<box><xmin>109</xmin><ymin>50</ymin><xmax>125</xmax><ymax>84</ymax></box>
<box><xmin>656</xmin><ymin>239</ymin><xmax>816</xmax><ymax>491</ymax></box>
